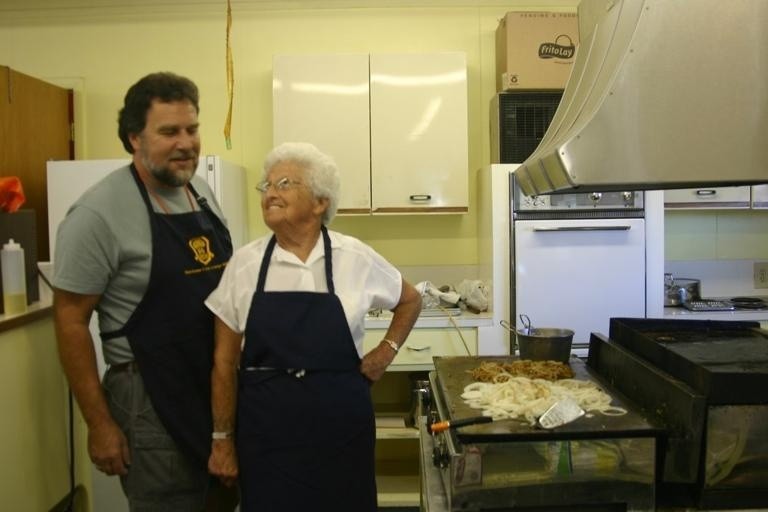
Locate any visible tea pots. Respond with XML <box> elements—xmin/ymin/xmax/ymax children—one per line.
<box><xmin>664</xmin><ymin>272</ymin><xmax>682</xmax><ymax>306</ymax></box>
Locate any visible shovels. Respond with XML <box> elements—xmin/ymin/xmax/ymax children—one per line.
<box><xmin>431</xmin><ymin>397</ymin><xmax>585</xmax><ymax>432</ymax></box>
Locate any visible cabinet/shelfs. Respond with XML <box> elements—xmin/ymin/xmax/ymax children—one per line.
<box><xmin>362</xmin><ymin>317</ymin><xmax>477</xmax><ymax>509</ymax></box>
<box><xmin>272</xmin><ymin>51</ymin><xmax>468</xmax><ymax>216</ymax></box>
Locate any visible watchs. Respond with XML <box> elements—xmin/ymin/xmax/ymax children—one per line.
<box><xmin>382</xmin><ymin>339</ymin><xmax>399</xmax><ymax>352</ymax></box>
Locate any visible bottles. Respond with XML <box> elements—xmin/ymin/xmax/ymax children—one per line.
<box><xmin>408</xmin><ymin>374</ymin><xmax>433</xmax><ymax>428</ymax></box>
<box><xmin>2</xmin><ymin>239</ymin><xmax>31</xmax><ymax>316</ymax></box>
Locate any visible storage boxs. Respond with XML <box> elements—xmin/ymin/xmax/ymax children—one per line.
<box><xmin>495</xmin><ymin>11</ymin><xmax>580</xmax><ymax>94</ymax></box>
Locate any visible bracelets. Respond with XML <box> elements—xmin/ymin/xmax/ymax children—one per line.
<box><xmin>211</xmin><ymin>431</ymin><xmax>235</xmax><ymax>440</ymax></box>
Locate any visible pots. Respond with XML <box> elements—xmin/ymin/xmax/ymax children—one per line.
<box><xmin>499</xmin><ymin>320</ymin><xmax>574</xmax><ymax>365</ymax></box>
<box><xmin>673</xmin><ymin>279</ymin><xmax>702</xmax><ymax>300</ymax></box>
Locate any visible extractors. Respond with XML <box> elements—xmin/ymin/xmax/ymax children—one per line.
<box><xmin>515</xmin><ymin>0</ymin><xmax>767</xmax><ymax>194</ymax></box>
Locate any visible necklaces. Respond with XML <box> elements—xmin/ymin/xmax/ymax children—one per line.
<box><xmin>148</xmin><ymin>184</ymin><xmax>196</xmax><ymax>215</ymax></box>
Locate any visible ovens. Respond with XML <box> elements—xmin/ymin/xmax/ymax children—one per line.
<box><xmin>469</xmin><ymin>165</ymin><xmax>666</xmax><ymax>361</ymax></box>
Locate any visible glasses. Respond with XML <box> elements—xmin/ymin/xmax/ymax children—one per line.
<box><xmin>256</xmin><ymin>177</ymin><xmax>301</xmax><ymax>192</ymax></box>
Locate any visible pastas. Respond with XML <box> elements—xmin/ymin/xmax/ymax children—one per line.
<box><xmin>471</xmin><ymin>359</ymin><xmax>573</xmax><ymax>381</ymax></box>
<box><xmin>461</xmin><ymin>374</ymin><xmax>627</xmax><ymax>427</ymax></box>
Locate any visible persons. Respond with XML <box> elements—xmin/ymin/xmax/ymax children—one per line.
<box><xmin>51</xmin><ymin>69</ymin><xmax>235</xmax><ymax>512</ymax></box>
<box><xmin>204</xmin><ymin>140</ymin><xmax>425</xmax><ymax>511</ymax></box>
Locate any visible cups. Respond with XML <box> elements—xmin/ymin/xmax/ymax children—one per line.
<box><xmin>37</xmin><ymin>260</ymin><xmax>53</xmax><ymax>304</ymax></box>
